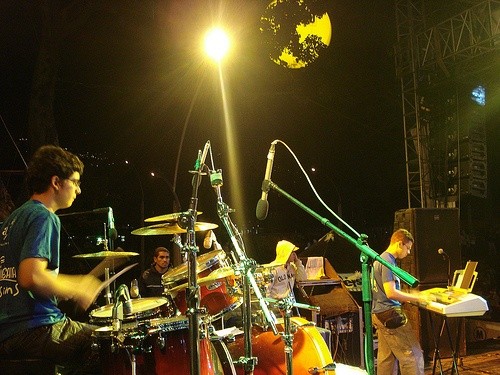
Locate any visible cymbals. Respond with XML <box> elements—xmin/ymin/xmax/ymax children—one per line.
<box><xmin>131</xmin><ymin>221</ymin><xmax>219</xmax><ymax>236</ymax></box>
<box><xmin>72</xmin><ymin>251</ymin><xmax>139</xmax><ymax>259</ymax></box>
<box><xmin>144</xmin><ymin>211</ymin><xmax>203</xmax><ymax>223</ymax></box>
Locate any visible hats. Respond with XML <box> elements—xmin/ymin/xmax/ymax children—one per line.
<box><xmin>293</xmin><ymin>246</ymin><xmax>299</xmax><ymax>251</ymax></box>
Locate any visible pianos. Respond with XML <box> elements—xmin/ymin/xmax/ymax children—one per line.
<box><xmin>408</xmin><ymin>286</ymin><xmax>490</xmax><ymax>375</ymax></box>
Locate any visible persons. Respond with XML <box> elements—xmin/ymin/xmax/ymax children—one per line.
<box><xmin>0</xmin><ymin>145</ymin><xmax>103</xmax><ymax>375</ymax></box>
<box><xmin>265</xmin><ymin>240</ymin><xmax>308</xmax><ymax>318</ymax></box>
<box><xmin>371</xmin><ymin>229</ymin><xmax>431</xmax><ymax>375</ymax></box>
<box><xmin>139</xmin><ymin>247</ymin><xmax>170</xmax><ymax>298</ymax></box>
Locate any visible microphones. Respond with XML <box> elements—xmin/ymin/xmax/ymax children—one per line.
<box><xmin>192</xmin><ymin>140</ymin><xmax>211</xmax><ymax>187</ymax></box>
<box><xmin>438</xmin><ymin>248</ymin><xmax>450</xmax><ymax>258</ymax></box>
<box><xmin>130</xmin><ymin>279</ymin><xmax>139</xmax><ymax>297</ymax></box>
<box><xmin>108</xmin><ymin>206</ymin><xmax>118</xmax><ymax>240</ymax></box>
<box><xmin>122</xmin><ymin>285</ymin><xmax>132</xmax><ymax>314</ymax></box>
<box><xmin>203</xmin><ymin>230</ymin><xmax>213</xmax><ymax>249</ymax></box>
<box><xmin>256</xmin><ymin>143</ymin><xmax>276</xmax><ymax>220</ymax></box>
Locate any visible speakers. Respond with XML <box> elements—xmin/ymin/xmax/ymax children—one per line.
<box><xmin>395</xmin><ymin>207</ymin><xmax>466</xmax><ymax>359</ymax></box>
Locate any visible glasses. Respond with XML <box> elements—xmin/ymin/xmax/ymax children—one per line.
<box><xmin>405</xmin><ymin>244</ymin><xmax>412</xmax><ymax>250</ymax></box>
<box><xmin>67</xmin><ymin>178</ymin><xmax>82</xmax><ymax>186</ymax></box>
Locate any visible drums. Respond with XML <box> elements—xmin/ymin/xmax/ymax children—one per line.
<box><xmin>89</xmin><ymin>298</ymin><xmax>173</xmax><ymax>363</ymax></box>
<box><xmin>230</xmin><ymin>263</ymin><xmax>274</xmax><ymax>284</ymax></box>
<box><xmin>210</xmin><ymin>315</ymin><xmax>338</xmax><ymax>375</ymax></box>
<box><xmin>91</xmin><ymin>313</ymin><xmax>212</xmax><ymax>375</ymax></box>
<box><xmin>162</xmin><ymin>250</ymin><xmax>244</xmax><ymax>321</ymax></box>
